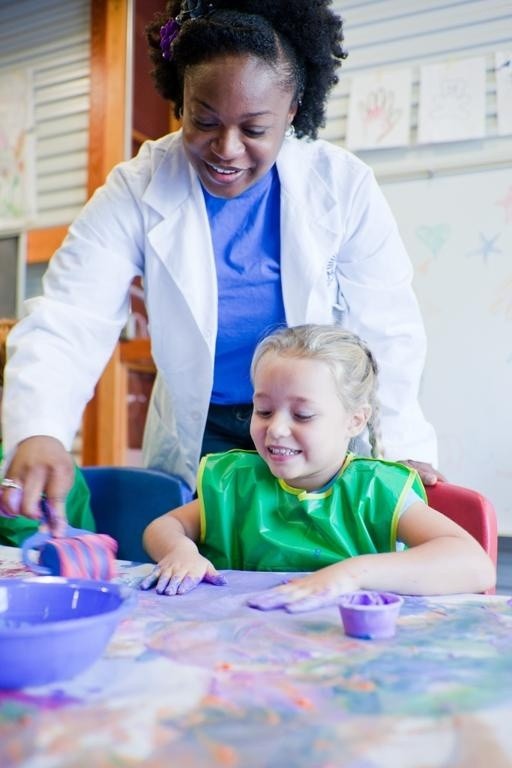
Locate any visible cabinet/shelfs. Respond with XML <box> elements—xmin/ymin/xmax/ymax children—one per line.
<box><xmin>84</xmin><ymin>339</ymin><xmax>157</xmax><ymax>465</ymax></box>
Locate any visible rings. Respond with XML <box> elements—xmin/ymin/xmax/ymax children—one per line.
<box><xmin>0</xmin><ymin>479</ymin><xmax>22</xmax><ymax>489</ymax></box>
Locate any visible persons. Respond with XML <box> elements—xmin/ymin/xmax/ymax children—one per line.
<box><xmin>141</xmin><ymin>322</ymin><xmax>497</xmax><ymax>614</ymax></box>
<box><xmin>0</xmin><ymin>1</ymin><xmax>445</xmax><ymax>541</ymax></box>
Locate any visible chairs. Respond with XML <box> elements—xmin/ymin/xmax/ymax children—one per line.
<box><xmin>427</xmin><ymin>483</ymin><xmax>499</xmax><ymax>594</ymax></box>
<box><xmin>77</xmin><ymin>467</ymin><xmax>194</xmax><ymax>564</ymax></box>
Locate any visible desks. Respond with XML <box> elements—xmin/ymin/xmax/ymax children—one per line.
<box><xmin>0</xmin><ymin>544</ymin><xmax>512</xmax><ymax>768</ymax></box>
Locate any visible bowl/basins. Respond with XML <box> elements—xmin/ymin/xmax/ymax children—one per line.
<box><xmin>0</xmin><ymin>575</ymin><xmax>138</xmax><ymax>693</ymax></box>
<box><xmin>336</xmin><ymin>591</ymin><xmax>402</xmax><ymax>639</ymax></box>
